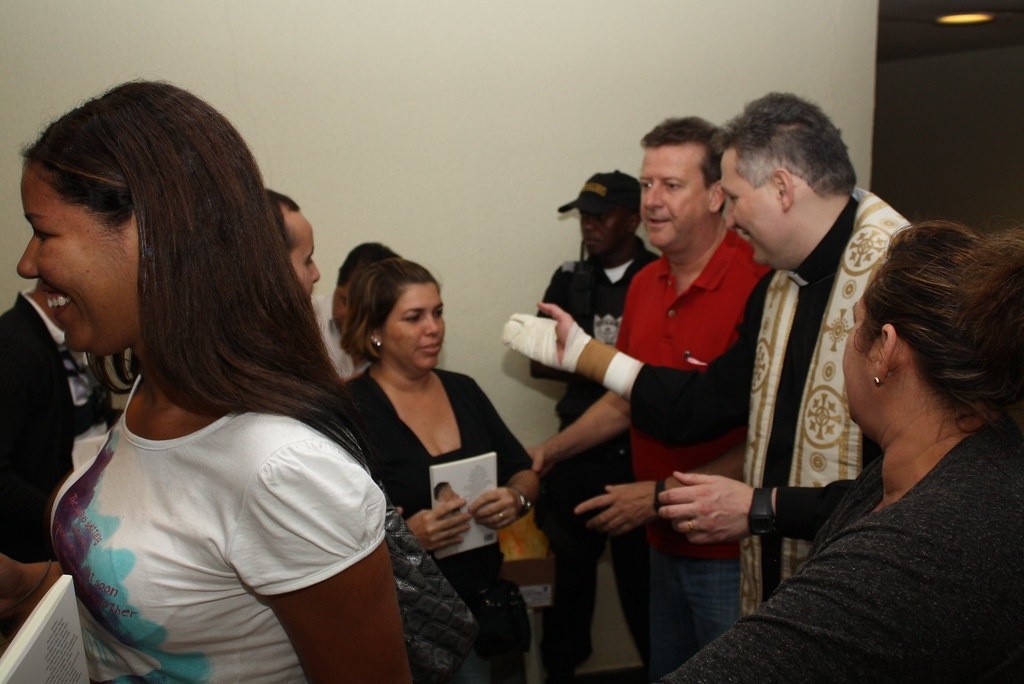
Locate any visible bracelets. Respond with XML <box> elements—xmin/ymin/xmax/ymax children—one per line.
<box><xmin>653</xmin><ymin>479</ymin><xmax>666</xmax><ymax>512</ymax></box>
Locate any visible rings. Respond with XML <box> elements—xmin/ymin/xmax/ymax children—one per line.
<box><xmin>688</xmin><ymin>520</ymin><xmax>695</xmax><ymax>532</ymax></box>
<box><xmin>498</xmin><ymin>512</ymin><xmax>504</xmax><ymax>519</ymax></box>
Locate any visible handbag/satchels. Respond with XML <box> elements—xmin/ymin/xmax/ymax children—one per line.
<box><xmin>375</xmin><ymin>480</ymin><xmax>481</xmax><ymax>684</ymax></box>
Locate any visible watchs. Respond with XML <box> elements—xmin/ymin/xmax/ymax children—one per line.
<box><xmin>749</xmin><ymin>488</ymin><xmax>775</xmax><ymax>535</ymax></box>
<box><xmin>519</xmin><ymin>494</ymin><xmax>532</xmax><ymax>517</ymax></box>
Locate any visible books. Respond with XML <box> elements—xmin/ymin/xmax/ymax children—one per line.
<box><xmin>429</xmin><ymin>452</ymin><xmax>498</xmax><ymax>560</ymax></box>
<box><xmin>0</xmin><ymin>575</ymin><xmax>91</xmax><ymax>684</ymax></box>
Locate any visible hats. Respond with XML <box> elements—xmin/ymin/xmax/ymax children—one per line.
<box><xmin>558</xmin><ymin>170</ymin><xmax>641</xmax><ymax>216</ymax></box>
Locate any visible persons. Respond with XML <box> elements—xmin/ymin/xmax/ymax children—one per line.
<box><xmin>658</xmin><ymin>217</ymin><xmax>1024</xmax><ymax>684</ymax></box>
<box><xmin>525</xmin><ymin>117</ymin><xmax>772</xmax><ymax>684</ymax></box>
<box><xmin>504</xmin><ymin>88</ymin><xmax>913</xmax><ymax>616</ymax></box>
<box><xmin>528</xmin><ymin>169</ymin><xmax>661</xmax><ymax>684</ymax></box>
<box><xmin>432</xmin><ymin>481</ymin><xmax>468</xmax><ymax>517</ymax></box>
<box><xmin>341</xmin><ymin>256</ymin><xmax>541</xmax><ymax>684</ymax></box>
<box><xmin>312</xmin><ymin>240</ymin><xmax>401</xmax><ymax>381</ymax></box>
<box><xmin>0</xmin><ymin>80</ymin><xmax>415</xmax><ymax>684</ymax></box>
<box><xmin>0</xmin><ymin>280</ymin><xmax>125</xmax><ymax>647</ymax></box>
<box><xmin>265</xmin><ymin>189</ymin><xmax>320</xmax><ymax>298</ymax></box>
